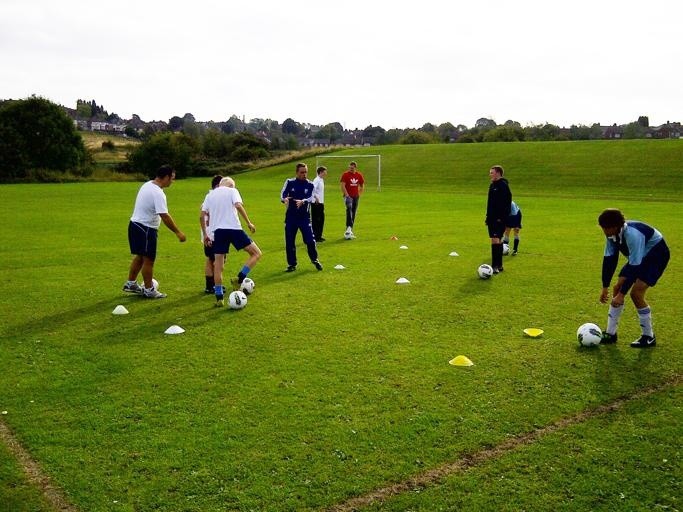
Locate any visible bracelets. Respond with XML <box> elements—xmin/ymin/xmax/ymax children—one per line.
<box><xmin>302</xmin><ymin>200</ymin><xmax>305</xmax><ymax>205</ymax></box>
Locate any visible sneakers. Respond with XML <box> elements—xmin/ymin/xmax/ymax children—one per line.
<box><xmin>600</xmin><ymin>330</ymin><xmax>619</xmax><ymax>343</ymax></box>
<box><xmin>283</xmin><ymin>265</ymin><xmax>297</xmax><ymax>272</ymax></box>
<box><xmin>204</xmin><ymin>277</ymin><xmax>241</xmax><ymax>307</ymax></box>
<box><xmin>630</xmin><ymin>333</ymin><xmax>656</xmax><ymax>349</ymax></box>
<box><xmin>123</xmin><ymin>280</ymin><xmax>167</xmax><ymax>298</ymax></box>
<box><xmin>310</xmin><ymin>259</ymin><xmax>323</xmax><ymax>270</ymax></box>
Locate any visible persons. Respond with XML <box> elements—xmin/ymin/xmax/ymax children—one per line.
<box><xmin>485</xmin><ymin>166</ymin><xmax>512</xmax><ymax>274</ymax></box>
<box><xmin>199</xmin><ymin>177</ymin><xmax>262</xmax><ymax>307</ymax></box>
<box><xmin>201</xmin><ymin>175</ymin><xmax>226</xmax><ymax>294</ymax></box>
<box><xmin>123</xmin><ymin>164</ymin><xmax>186</xmax><ymax>298</ymax></box>
<box><xmin>280</xmin><ymin>163</ymin><xmax>323</xmax><ymax>272</ymax></box>
<box><xmin>340</xmin><ymin>162</ymin><xmax>364</xmax><ymax>230</ymax></box>
<box><xmin>599</xmin><ymin>208</ymin><xmax>670</xmax><ymax>348</ymax></box>
<box><xmin>311</xmin><ymin>166</ymin><xmax>328</xmax><ymax>242</ymax></box>
<box><xmin>503</xmin><ymin>201</ymin><xmax>522</xmax><ymax>256</ymax></box>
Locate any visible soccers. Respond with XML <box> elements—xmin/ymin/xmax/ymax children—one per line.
<box><xmin>229</xmin><ymin>290</ymin><xmax>248</xmax><ymax>310</ymax></box>
<box><xmin>502</xmin><ymin>244</ymin><xmax>510</xmax><ymax>254</ymax></box>
<box><xmin>240</xmin><ymin>278</ymin><xmax>254</xmax><ymax>295</ymax></box>
<box><xmin>577</xmin><ymin>323</ymin><xmax>602</xmax><ymax>346</ymax></box>
<box><xmin>478</xmin><ymin>264</ymin><xmax>494</xmax><ymax>279</ymax></box>
<box><xmin>343</xmin><ymin>230</ymin><xmax>353</xmax><ymax>240</ymax></box>
<box><xmin>141</xmin><ymin>278</ymin><xmax>159</xmax><ymax>293</ymax></box>
